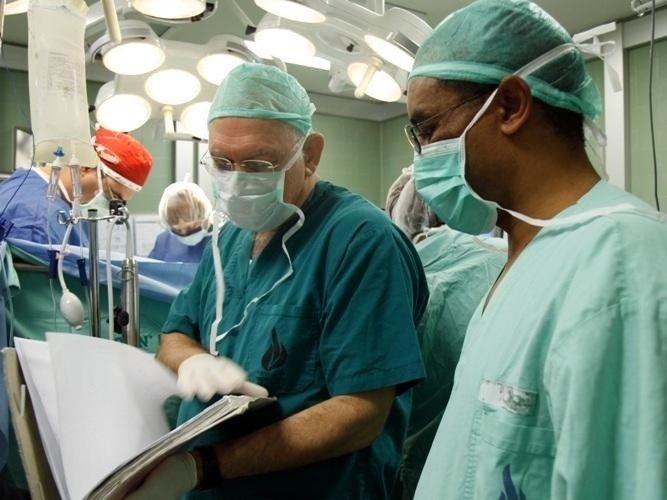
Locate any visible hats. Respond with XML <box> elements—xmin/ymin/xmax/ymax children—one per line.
<box><xmin>93</xmin><ymin>128</ymin><xmax>153</xmax><ymax>192</ymax></box>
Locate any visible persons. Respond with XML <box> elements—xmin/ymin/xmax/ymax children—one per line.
<box><xmin>1</xmin><ymin>126</ymin><xmax>521</xmax><ymax>500</ymax></box>
<box><xmin>401</xmin><ymin>0</ymin><xmax>665</xmax><ymax>500</ymax></box>
<box><xmin>152</xmin><ymin>61</ymin><xmax>431</xmax><ymax>500</ymax></box>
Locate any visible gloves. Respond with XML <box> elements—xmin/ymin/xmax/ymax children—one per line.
<box><xmin>175</xmin><ymin>353</ymin><xmax>269</xmax><ymax>404</ymax></box>
<box><xmin>124</xmin><ymin>449</ymin><xmax>198</xmax><ymax>500</ymax></box>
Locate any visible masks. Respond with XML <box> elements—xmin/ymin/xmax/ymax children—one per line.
<box><xmin>170</xmin><ymin>230</ymin><xmax>204</xmax><ymax>246</ymax></box>
<box><xmin>210</xmin><ymin>167</ymin><xmax>295</xmax><ymax>232</ymax></box>
<box><xmin>72</xmin><ymin>193</ymin><xmax>110</xmax><ymax>220</ymax></box>
<box><xmin>413</xmin><ymin>137</ymin><xmax>498</xmax><ymax>234</ymax></box>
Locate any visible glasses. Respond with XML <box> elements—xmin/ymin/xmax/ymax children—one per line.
<box><xmin>100</xmin><ymin>169</ymin><xmax>120</xmax><ymax>200</ymax></box>
<box><xmin>404</xmin><ymin>88</ymin><xmax>489</xmax><ymax>153</ymax></box>
<box><xmin>200</xmin><ymin>136</ymin><xmax>304</xmax><ymax>178</ymax></box>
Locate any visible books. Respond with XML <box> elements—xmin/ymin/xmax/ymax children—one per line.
<box><xmin>1</xmin><ymin>330</ymin><xmax>278</xmax><ymax>500</ymax></box>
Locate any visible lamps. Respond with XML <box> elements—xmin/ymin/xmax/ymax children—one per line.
<box><xmin>4</xmin><ymin>0</ymin><xmax>435</xmax><ymax>158</ymax></box>
